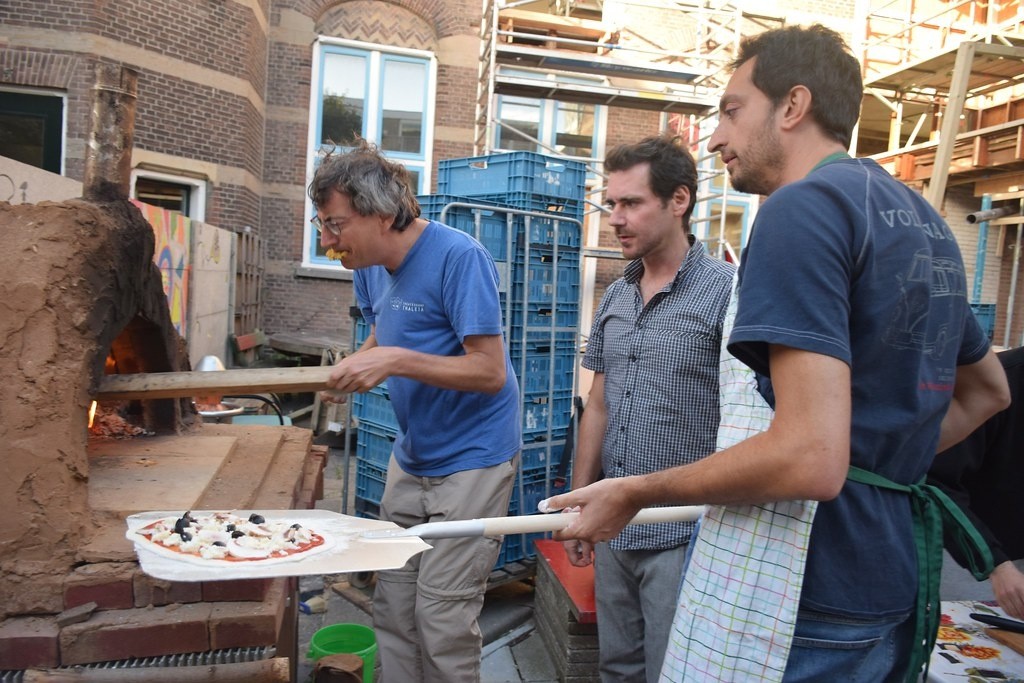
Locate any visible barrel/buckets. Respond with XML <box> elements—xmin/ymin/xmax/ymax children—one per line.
<box><xmin>306</xmin><ymin>623</ymin><xmax>379</xmax><ymax>683</ymax></box>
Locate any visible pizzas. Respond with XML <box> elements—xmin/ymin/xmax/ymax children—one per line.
<box><xmin>124</xmin><ymin>512</ymin><xmax>336</xmax><ymax>566</ymax></box>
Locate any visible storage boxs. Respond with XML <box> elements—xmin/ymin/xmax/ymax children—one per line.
<box><xmin>353</xmin><ymin>151</ymin><xmax>587</xmax><ymax>575</ymax></box>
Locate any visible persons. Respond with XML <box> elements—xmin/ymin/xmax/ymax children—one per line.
<box><xmin>308</xmin><ymin>146</ymin><xmax>522</xmax><ymax>683</ymax></box>
<box><xmin>563</xmin><ymin>134</ymin><xmax>737</xmax><ymax>683</ymax></box>
<box><xmin>934</xmin><ymin>346</ymin><xmax>1024</xmax><ymax>621</ymax></box>
<box><xmin>537</xmin><ymin>23</ymin><xmax>1011</xmax><ymax>683</ymax></box>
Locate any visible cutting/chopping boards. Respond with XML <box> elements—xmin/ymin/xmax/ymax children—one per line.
<box><xmin>984</xmin><ymin>627</ymin><xmax>1024</xmax><ymax>656</ymax></box>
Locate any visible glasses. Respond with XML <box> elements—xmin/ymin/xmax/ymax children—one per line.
<box><xmin>309</xmin><ymin>213</ymin><xmax>361</xmax><ymax>236</ymax></box>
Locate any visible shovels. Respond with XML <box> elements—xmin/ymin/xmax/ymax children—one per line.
<box><xmin>123</xmin><ymin>495</ymin><xmax>715</xmax><ymax>584</ymax></box>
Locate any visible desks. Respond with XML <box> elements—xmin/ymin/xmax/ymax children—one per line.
<box><xmin>919</xmin><ymin>599</ymin><xmax>1024</xmax><ymax>683</ymax></box>
